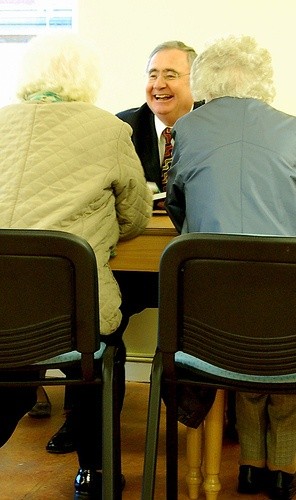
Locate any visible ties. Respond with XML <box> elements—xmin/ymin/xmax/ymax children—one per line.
<box><xmin>161</xmin><ymin>128</ymin><xmax>174</xmax><ymax>191</ymax></box>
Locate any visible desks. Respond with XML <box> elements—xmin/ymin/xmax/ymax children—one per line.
<box><xmin>108</xmin><ymin>217</ymin><xmax>225</xmax><ymax>500</ymax></box>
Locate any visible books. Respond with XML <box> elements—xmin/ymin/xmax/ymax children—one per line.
<box><xmin>152</xmin><ymin>192</ymin><xmax>167</xmax><ymax>200</ymax></box>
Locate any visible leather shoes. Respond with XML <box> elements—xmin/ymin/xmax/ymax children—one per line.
<box><xmin>46</xmin><ymin>421</ymin><xmax>76</xmax><ymax>454</ymax></box>
<box><xmin>238</xmin><ymin>464</ymin><xmax>296</xmax><ymax>500</ymax></box>
<box><xmin>73</xmin><ymin>468</ymin><xmax>125</xmax><ymax>500</ymax></box>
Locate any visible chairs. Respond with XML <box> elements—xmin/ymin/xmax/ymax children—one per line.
<box><xmin>142</xmin><ymin>232</ymin><xmax>296</xmax><ymax>500</ymax></box>
<box><xmin>0</xmin><ymin>228</ymin><xmax>113</xmax><ymax>500</ymax></box>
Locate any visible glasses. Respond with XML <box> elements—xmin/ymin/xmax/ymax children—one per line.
<box><xmin>147</xmin><ymin>71</ymin><xmax>191</xmax><ymax>82</ymax></box>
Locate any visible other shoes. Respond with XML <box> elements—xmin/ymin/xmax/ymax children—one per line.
<box><xmin>28</xmin><ymin>387</ymin><xmax>52</xmax><ymax>417</ymax></box>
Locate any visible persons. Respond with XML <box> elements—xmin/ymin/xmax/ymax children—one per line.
<box><xmin>1</xmin><ymin>30</ymin><xmax>153</xmax><ymax>500</ymax></box>
<box><xmin>29</xmin><ymin>387</ymin><xmax>52</xmax><ymax>417</ymax></box>
<box><xmin>165</xmin><ymin>36</ymin><xmax>296</xmax><ymax>500</ymax></box>
<box><xmin>46</xmin><ymin>40</ymin><xmax>240</xmax><ymax>454</ymax></box>
